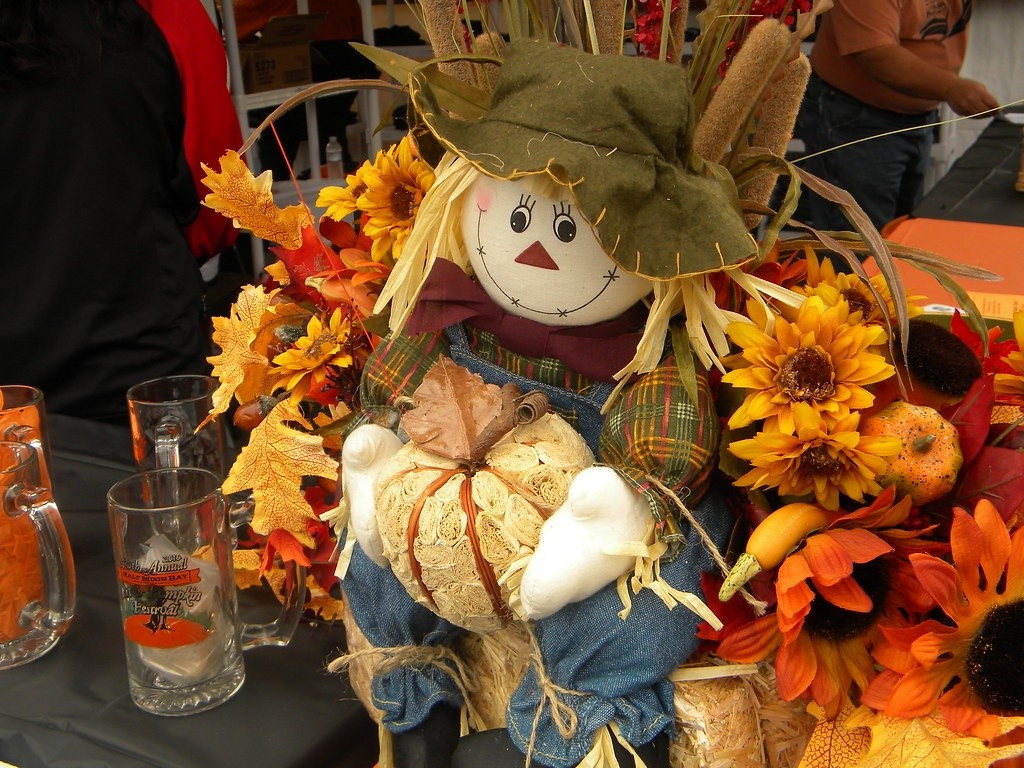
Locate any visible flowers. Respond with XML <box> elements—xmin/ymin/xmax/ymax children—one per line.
<box><xmin>189</xmin><ymin>130</ymin><xmax>439</xmax><ymax>624</ymax></box>
<box><xmin>705</xmin><ymin>247</ymin><xmax>1024</xmax><ymax>768</ymax></box>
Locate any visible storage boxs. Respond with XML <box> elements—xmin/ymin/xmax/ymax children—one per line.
<box><xmin>240</xmin><ymin>12</ymin><xmax>326</xmax><ymax>93</ymax></box>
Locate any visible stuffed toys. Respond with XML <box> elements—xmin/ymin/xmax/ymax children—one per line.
<box><xmin>320</xmin><ymin>35</ymin><xmax>759</xmax><ymax>768</ymax></box>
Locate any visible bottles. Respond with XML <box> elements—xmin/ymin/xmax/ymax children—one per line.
<box><xmin>326</xmin><ymin>137</ymin><xmax>343</xmax><ymax>185</ymax></box>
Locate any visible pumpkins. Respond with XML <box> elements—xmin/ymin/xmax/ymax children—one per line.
<box><xmin>863</xmin><ymin>401</ymin><xmax>962</xmax><ymax>504</ymax></box>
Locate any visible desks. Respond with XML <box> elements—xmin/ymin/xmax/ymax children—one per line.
<box><xmin>0</xmin><ymin>412</ymin><xmax>380</xmax><ymax>768</ymax></box>
<box><xmin>852</xmin><ymin>216</ymin><xmax>1024</xmax><ymax>320</ymax></box>
<box><xmin>909</xmin><ymin>105</ymin><xmax>1024</xmax><ymax>227</ymax></box>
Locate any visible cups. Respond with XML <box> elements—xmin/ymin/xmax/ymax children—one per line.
<box><xmin>0</xmin><ymin>441</ymin><xmax>74</xmax><ymax>670</ymax></box>
<box><xmin>106</xmin><ymin>468</ymin><xmax>305</xmax><ymax>717</ymax></box>
<box><xmin>0</xmin><ymin>385</ymin><xmax>52</xmax><ymax>499</ymax></box>
<box><xmin>127</xmin><ymin>374</ymin><xmax>224</xmax><ymax>479</ymax></box>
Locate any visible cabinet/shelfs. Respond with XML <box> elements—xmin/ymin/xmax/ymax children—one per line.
<box><xmin>212</xmin><ymin>0</ymin><xmax>381</xmax><ymax>282</ymax></box>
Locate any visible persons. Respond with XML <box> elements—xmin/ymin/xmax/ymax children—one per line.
<box><xmin>799</xmin><ymin>0</ymin><xmax>1001</xmax><ymax>235</ymax></box>
<box><xmin>0</xmin><ymin>0</ymin><xmax>248</xmax><ymax>427</ymax></box>
